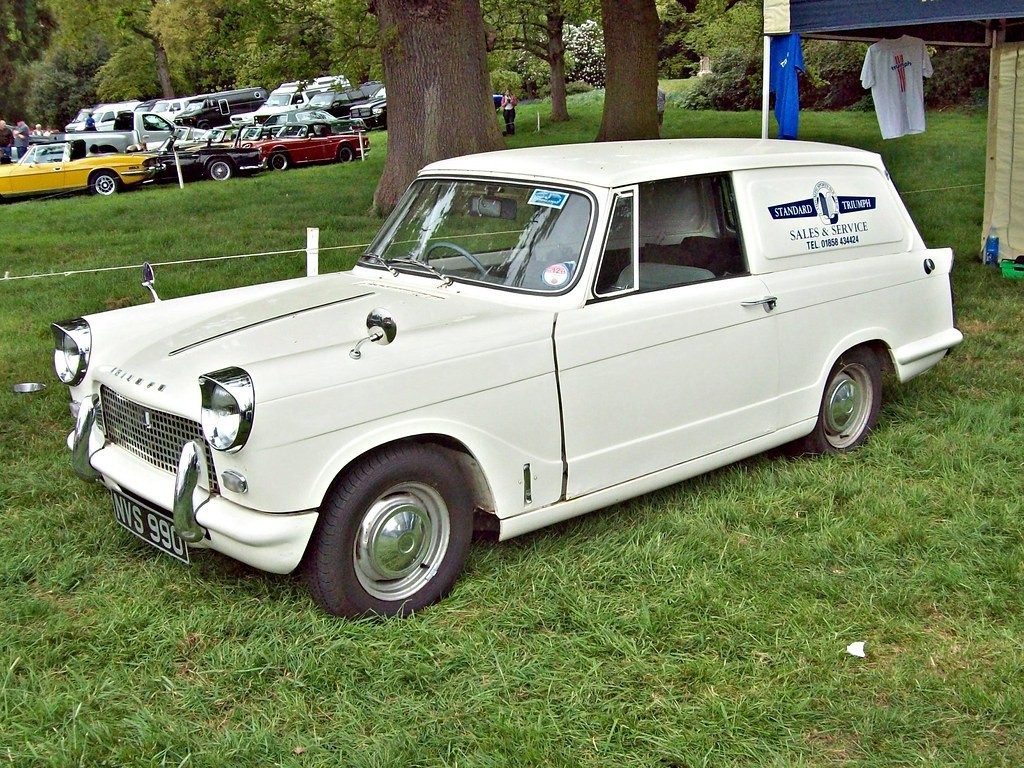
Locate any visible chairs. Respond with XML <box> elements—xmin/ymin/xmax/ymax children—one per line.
<box><xmin>604</xmin><ymin>262</ymin><xmax>717</xmax><ymax>295</ymax></box>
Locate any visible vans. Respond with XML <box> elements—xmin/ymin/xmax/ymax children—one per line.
<box><xmin>174</xmin><ymin>87</ymin><xmax>269</xmax><ymax>131</ymax></box>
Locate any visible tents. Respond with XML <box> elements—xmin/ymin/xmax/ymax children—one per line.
<box><xmin>761</xmin><ymin>0</ymin><xmax>1024</xmax><ymax>262</ymax></box>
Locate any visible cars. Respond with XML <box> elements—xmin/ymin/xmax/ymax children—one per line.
<box><xmin>50</xmin><ymin>140</ymin><xmax>963</xmax><ymax>630</ymax></box>
<box><xmin>0</xmin><ymin>140</ymin><xmax>159</xmax><ymax>198</ymax></box>
<box><xmin>26</xmin><ymin>74</ymin><xmax>387</xmax><ymax>184</ymax></box>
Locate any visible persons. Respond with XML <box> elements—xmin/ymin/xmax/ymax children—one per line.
<box><xmin>0</xmin><ymin>119</ymin><xmax>14</xmax><ymax>157</ymax></box>
<box><xmin>501</xmin><ymin>88</ymin><xmax>517</xmax><ymax>135</ymax></box>
<box><xmin>12</xmin><ymin>118</ymin><xmax>29</xmax><ymax>159</ymax></box>
<box><xmin>85</xmin><ymin>113</ymin><xmax>95</xmax><ymax>127</ymax></box>
<box><xmin>32</xmin><ymin>123</ymin><xmax>53</xmax><ymax>136</ymax></box>
<box><xmin>656</xmin><ymin>81</ymin><xmax>666</xmax><ymax>136</ymax></box>
